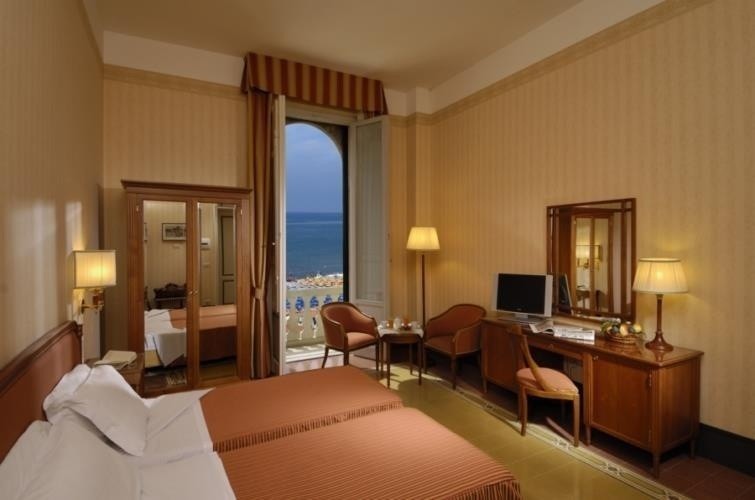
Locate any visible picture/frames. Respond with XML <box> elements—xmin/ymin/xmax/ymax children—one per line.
<box><xmin>161</xmin><ymin>222</ymin><xmax>188</xmax><ymax>241</ymax></box>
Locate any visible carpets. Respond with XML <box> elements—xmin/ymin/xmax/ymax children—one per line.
<box><xmin>368</xmin><ymin>356</ymin><xmax>687</xmax><ymax>499</ymax></box>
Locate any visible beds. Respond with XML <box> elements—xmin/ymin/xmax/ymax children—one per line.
<box><xmin>143</xmin><ymin>302</ymin><xmax>240</xmax><ymax>369</ymax></box>
<box><xmin>0</xmin><ymin>318</ymin><xmax>525</xmax><ymax>500</ymax></box>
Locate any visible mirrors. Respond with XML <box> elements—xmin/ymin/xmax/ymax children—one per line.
<box><xmin>542</xmin><ymin>194</ymin><xmax>640</xmax><ymax>329</ymax></box>
<box><xmin>135</xmin><ymin>197</ymin><xmax>238</xmax><ymax>394</ymax></box>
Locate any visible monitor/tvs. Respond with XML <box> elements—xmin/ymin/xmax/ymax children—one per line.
<box><xmin>491</xmin><ymin>272</ymin><xmax>553</xmax><ymax>323</ymax></box>
<box><xmin>560</xmin><ymin>273</ymin><xmax>573</xmax><ymax>314</ymax></box>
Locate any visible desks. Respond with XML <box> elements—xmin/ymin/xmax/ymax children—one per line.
<box><xmin>477</xmin><ymin>304</ymin><xmax>707</xmax><ymax>479</ymax></box>
<box><xmin>376</xmin><ymin>322</ymin><xmax>422</xmax><ymax>391</ymax></box>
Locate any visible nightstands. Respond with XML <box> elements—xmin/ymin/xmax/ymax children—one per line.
<box><xmin>81</xmin><ymin>348</ymin><xmax>146</xmax><ymax>398</ymax></box>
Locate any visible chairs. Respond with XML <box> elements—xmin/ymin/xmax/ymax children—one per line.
<box><xmin>511</xmin><ymin>323</ymin><xmax>581</xmax><ymax>449</ymax></box>
<box><xmin>152</xmin><ymin>281</ymin><xmax>186</xmax><ymax>310</ymax></box>
<box><xmin>423</xmin><ymin>302</ymin><xmax>489</xmax><ymax>391</ymax></box>
<box><xmin>320</xmin><ymin>301</ymin><xmax>380</xmax><ymax>379</ymax></box>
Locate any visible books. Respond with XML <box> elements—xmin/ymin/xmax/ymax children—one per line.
<box><xmin>529</xmin><ymin>320</ymin><xmax>596</xmax><ymax>341</ymax></box>
<box><xmin>93</xmin><ymin>350</ymin><xmax>138</xmax><ymax>371</ymax></box>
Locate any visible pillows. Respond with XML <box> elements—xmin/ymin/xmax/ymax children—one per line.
<box><xmin>42</xmin><ymin>361</ymin><xmax>102</xmax><ymax>443</ymax></box>
<box><xmin>0</xmin><ymin>410</ymin><xmax>45</xmax><ymax>499</ymax></box>
<box><xmin>64</xmin><ymin>362</ymin><xmax>152</xmax><ymax>456</ymax></box>
<box><xmin>8</xmin><ymin>420</ymin><xmax>144</xmax><ymax>499</ymax></box>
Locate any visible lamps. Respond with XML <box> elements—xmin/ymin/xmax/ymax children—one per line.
<box><xmin>405</xmin><ymin>223</ymin><xmax>442</xmax><ymax>322</ymax></box>
<box><xmin>70</xmin><ymin>247</ymin><xmax>118</xmax><ymax>313</ymax></box>
<box><xmin>632</xmin><ymin>255</ymin><xmax>692</xmax><ymax>354</ymax></box>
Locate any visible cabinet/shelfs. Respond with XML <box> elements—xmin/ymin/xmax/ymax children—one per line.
<box><xmin>116</xmin><ymin>175</ymin><xmax>256</xmax><ymax>399</ymax></box>
<box><xmin>576</xmin><ymin>288</ymin><xmax>600</xmax><ymax>311</ymax></box>
